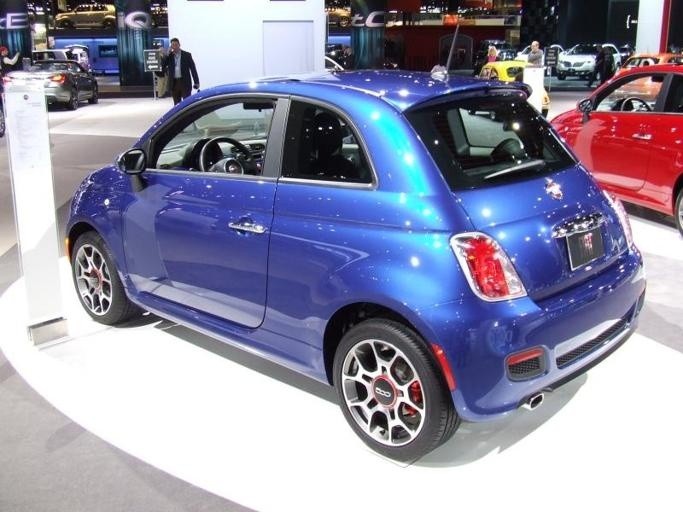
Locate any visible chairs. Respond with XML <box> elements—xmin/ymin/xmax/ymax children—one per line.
<box><xmin>305</xmin><ymin>112</ymin><xmax>359</xmax><ymax>180</ymax></box>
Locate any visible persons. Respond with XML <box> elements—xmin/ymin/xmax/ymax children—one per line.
<box><xmin>528</xmin><ymin>40</ymin><xmax>543</xmax><ymax>65</ymax></box>
<box><xmin>603</xmin><ymin>47</ymin><xmax>615</xmax><ymax>80</ymax></box>
<box><xmin>584</xmin><ymin>46</ymin><xmax>605</xmax><ymax>88</ymax></box>
<box><xmin>0</xmin><ymin>45</ymin><xmax>21</xmax><ymax>76</ymax></box>
<box><xmin>165</xmin><ymin>39</ymin><xmax>199</xmax><ymax>106</ymax></box>
<box><xmin>643</xmin><ymin>61</ymin><xmax>649</xmax><ymax>66</ymax></box>
<box><xmin>155</xmin><ymin>47</ymin><xmax>169</xmax><ymax>98</ymax></box>
<box><xmin>487</xmin><ymin>45</ymin><xmax>497</xmax><ymax>62</ymax></box>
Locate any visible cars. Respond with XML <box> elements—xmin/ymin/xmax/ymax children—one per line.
<box><xmin>548</xmin><ymin>62</ymin><xmax>683</xmax><ymax>239</ymax></box>
<box><xmin>2</xmin><ymin>44</ymin><xmax>98</xmax><ymax>110</ymax></box>
<box><xmin>64</xmin><ymin>65</ymin><xmax>649</xmax><ymax>461</ymax></box>
<box><xmin>325</xmin><ymin>6</ymin><xmax>351</xmax><ymax>28</ymax></box>
<box><xmin>485</xmin><ymin>39</ymin><xmax>633</xmax><ymax>80</ymax></box>
<box><xmin>55</xmin><ymin>4</ymin><xmax>116</xmax><ymax>31</ymax></box>
<box><xmin>479</xmin><ymin>58</ymin><xmax>529</xmax><ymax>83</ymax></box>
<box><xmin>613</xmin><ymin>53</ymin><xmax>683</xmax><ymax>102</ymax></box>
